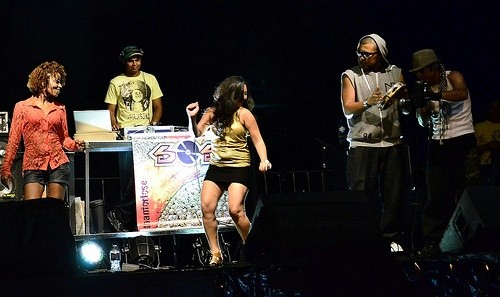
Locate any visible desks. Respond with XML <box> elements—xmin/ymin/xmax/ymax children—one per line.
<box><xmin>74</xmin><ymin>139</ymin><xmax>133</xmax><ymax>234</ymax></box>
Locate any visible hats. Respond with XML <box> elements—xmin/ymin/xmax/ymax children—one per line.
<box><xmin>119</xmin><ymin>45</ymin><xmax>144</xmax><ymax>62</ymax></box>
<box><xmin>408</xmin><ymin>49</ymin><xmax>438</xmax><ymax>72</ymax></box>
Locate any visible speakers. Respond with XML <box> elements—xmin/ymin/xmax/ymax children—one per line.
<box><xmin>0</xmin><ymin>197</ymin><xmax>77</xmax><ymax>278</ymax></box>
<box><xmin>240</xmin><ymin>191</ymin><xmax>383</xmax><ymax>265</ymax></box>
<box><xmin>438</xmin><ymin>186</ymin><xmax>500</xmax><ymax>254</ymax></box>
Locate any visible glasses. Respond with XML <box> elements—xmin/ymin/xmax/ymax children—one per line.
<box><xmin>355</xmin><ymin>50</ymin><xmax>379</xmax><ymax>59</ymax></box>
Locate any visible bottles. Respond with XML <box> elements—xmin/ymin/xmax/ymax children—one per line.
<box><xmin>110</xmin><ymin>245</ymin><xmax>121</xmax><ymax>271</ymax></box>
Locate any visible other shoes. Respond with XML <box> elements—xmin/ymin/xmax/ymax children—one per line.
<box><xmin>209</xmin><ymin>251</ymin><xmax>223</xmax><ymax>266</ymax></box>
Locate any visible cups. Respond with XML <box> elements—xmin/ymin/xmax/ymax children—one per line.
<box><xmin>144</xmin><ymin>127</ymin><xmax>155</xmax><ymax>139</ymax></box>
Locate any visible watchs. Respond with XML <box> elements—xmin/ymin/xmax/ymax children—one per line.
<box><xmin>362</xmin><ymin>98</ymin><xmax>372</xmax><ymax>109</ymax></box>
<box><xmin>264</xmin><ymin>160</ymin><xmax>271</xmax><ymax>164</ymax></box>
<box><xmin>150</xmin><ymin>120</ymin><xmax>158</xmax><ymax>127</ymax></box>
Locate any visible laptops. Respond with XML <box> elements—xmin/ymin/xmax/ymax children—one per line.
<box><xmin>73</xmin><ymin>110</ymin><xmax>120</xmax><ymax>133</ymax></box>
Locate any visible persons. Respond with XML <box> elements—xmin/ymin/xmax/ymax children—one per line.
<box><xmin>105</xmin><ymin>46</ymin><xmax>163</xmax><ymax>231</ymax></box>
<box><xmin>391</xmin><ymin>48</ymin><xmax>500</xmax><ymax>262</ymax></box>
<box><xmin>185</xmin><ymin>76</ymin><xmax>273</xmax><ymax>264</ymax></box>
<box><xmin>340</xmin><ymin>33</ymin><xmax>411</xmax><ymax>239</ymax></box>
<box><xmin>1</xmin><ymin>61</ymin><xmax>86</xmax><ymax>201</ymax></box>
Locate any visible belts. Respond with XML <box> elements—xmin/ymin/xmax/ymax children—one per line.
<box><xmin>432</xmin><ymin>135</ymin><xmax>467</xmax><ymax>146</ymax></box>
